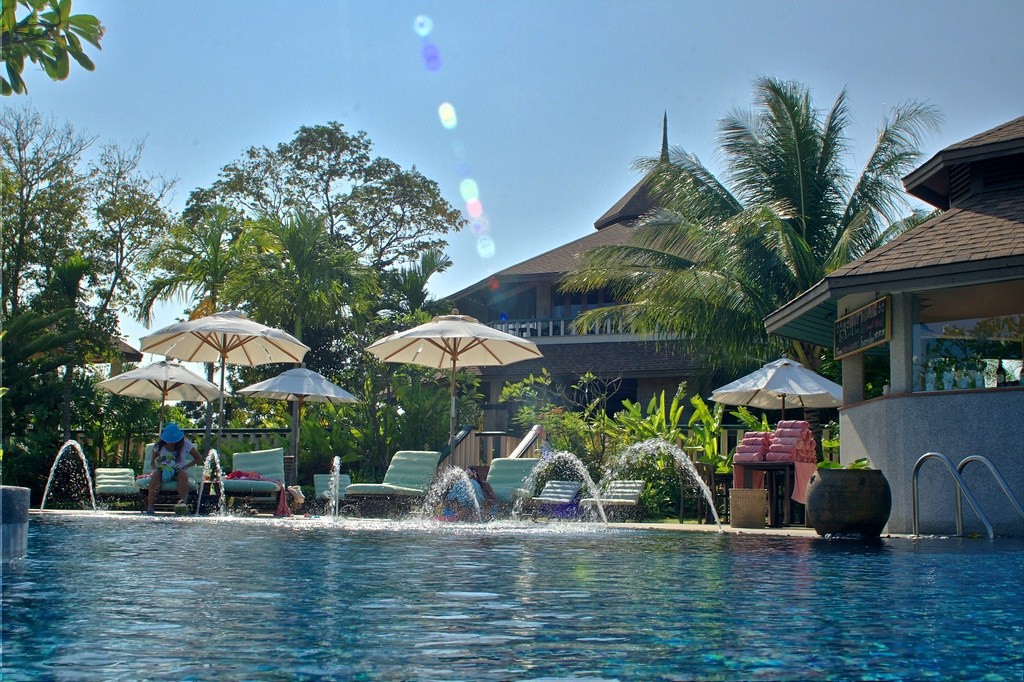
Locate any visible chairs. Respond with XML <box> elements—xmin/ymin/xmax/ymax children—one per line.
<box><xmin>224</xmin><ymin>446</ymin><xmax>287</xmax><ymax>512</ymax></box>
<box><xmin>678</xmin><ymin>462</ymin><xmax>729</xmax><ymax>524</ymax></box>
<box><xmin>531</xmin><ymin>480</ymin><xmax>582</xmax><ymax>520</ymax></box>
<box><xmin>483</xmin><ymin>458</ymin><xmax>541</xmax><ymax>504</ymax></box>
<box><xmin>315</xmin><ymin>474</ymin><xmax>352</xmax><ymax>515</ymax></box>
<box><xmin>346</xmin><ymin>450</ymin><xmax>441</xmax><ymax>518</ymax></box>
<box><xmin>94</xmin><ymin>467</ymin><xmax>141</xmax><ymax>508</ymax></box>
<box><xmin>138</xmin><ymin>443</ymin><xmax>198</xmax><ymax>508</ymax></box>
<box><xmin>580</xmin><ymin>480</ymin><xmax>646</xmax><ymax>522</ymax></box>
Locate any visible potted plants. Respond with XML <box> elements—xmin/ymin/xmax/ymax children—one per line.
<box><xmin>805</xmin><ymin>458</ymin><xmax>892</xmax><ymax>538</ymax></box>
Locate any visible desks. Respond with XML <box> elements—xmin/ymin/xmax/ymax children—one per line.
<box><xmin>733</xmin><ymin>460</ymin><xmax>800</xmax><ymax>527</ymax></box>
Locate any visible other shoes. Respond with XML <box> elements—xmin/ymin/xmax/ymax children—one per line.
<box><xmin>147</xmin><ymin>507</ymin><xmax>154</xmax><ymax>514</ymax></box>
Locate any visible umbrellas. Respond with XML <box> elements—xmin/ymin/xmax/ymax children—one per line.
<box><xmin>707</xmin><ymin>354</ymin><xmax>843</xmax><ymax>419</ymax></box>
<box><xmin>139</xmin><ymin>305</ymin><xmax>311</xmax><ymax>454</ymax></box>
<box><xmin>364</xmin><ymin>309</ymin><xmax>543</xmax><ymax>467</ymax></box>
<box><xmin>96</xmin><ymin>356</ymin><xmax>231</xmax><ymax>435</ymax></box>
<box><xmin>236</xmin><ymin>363</ymin><xmax>360</xmax><ymax>485</ymax></box>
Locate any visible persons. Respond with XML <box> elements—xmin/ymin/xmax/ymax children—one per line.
<box><xmin>447</xmin><ymin>468</ymin><xmax>484</xmax><ymax>517</ymax></box>
<box><xmin>140</xmin><ymin>424</ymin><xmax>203</xmax><ymax>516</ymax></box>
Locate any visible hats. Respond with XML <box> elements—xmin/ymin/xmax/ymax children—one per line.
<box><xmin>161</xmin><ymin>424</ymin><xmax>184</xmax><ymax>444</ymax></box>
